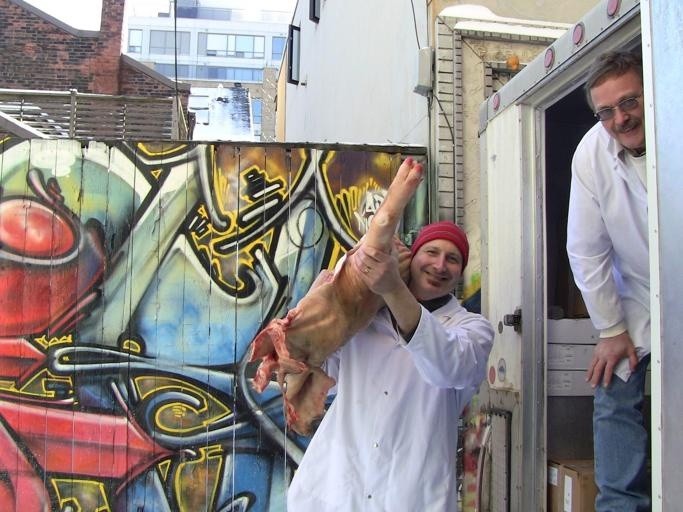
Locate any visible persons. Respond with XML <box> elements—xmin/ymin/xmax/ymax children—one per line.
<box><xmin>286</xmin><ymin>221</ymin><xmax>496</xmax><ymax>512</ymax></box>
<box><xmin>563</xmin><ymin>47</ymin><xmax>651</xmax><ymax>512</ymax></box>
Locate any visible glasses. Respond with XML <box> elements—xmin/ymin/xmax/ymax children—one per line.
<box><xmin>595</xmin><ymin>96</ymin><xmax>640</xmax><ymax>121</ymax></box>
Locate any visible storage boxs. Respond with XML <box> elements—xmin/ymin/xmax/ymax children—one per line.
<box><xmin>546</xmin><ymin>456</ymin><xmax>597</xmax><ymax>512</ymax></box>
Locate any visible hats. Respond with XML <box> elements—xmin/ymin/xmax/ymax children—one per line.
<box><xmin>410</xmin><ymin>221</ymin><xmax>469</xmax><ymax>274</ymax></box>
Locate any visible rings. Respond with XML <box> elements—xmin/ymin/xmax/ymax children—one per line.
<box><xmin>364</xmin><ymin>267</ymin><xmax>370</xmax><ymax>273</ymax></box>
